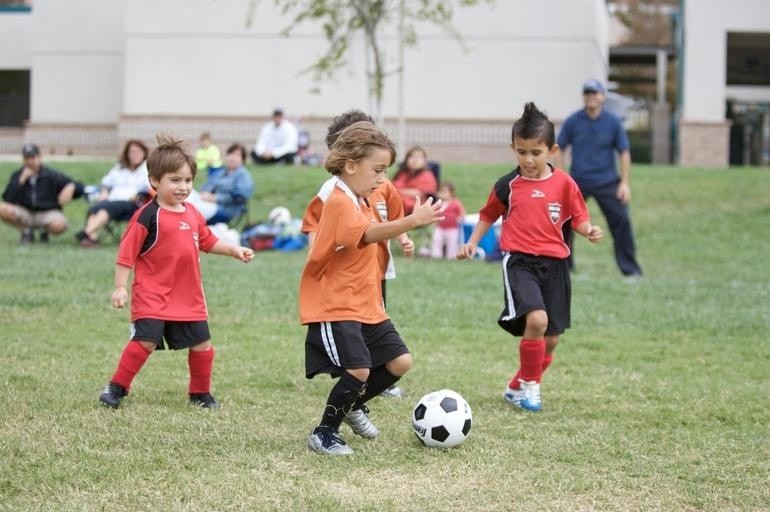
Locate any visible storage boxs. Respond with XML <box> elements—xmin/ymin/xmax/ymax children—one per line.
<box><xmin>458</xmin><ymin>213</ymin><xmax>503</xmax><ymax>261</ymax></box>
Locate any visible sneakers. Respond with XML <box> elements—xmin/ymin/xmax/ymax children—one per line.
<box><xmin>101</xmin><ymin>381</ymin><xmax>127</xmax><ymax>407</ymax></box>
<box><xmin>382</xmin><ymin>385</ymin><xmax>400</xmax><ymax>398</ymax></box>
<box><xmin>189</xmin><ymin>393</ymin><xmax>218</xmax><ymax>410</ymax></box>
<box><xmin>505</xmin><ymin>386</ymin><xmax>524</xmax><ymax>406</ymax></box>
<box><xmin>342</xmin><ymin>410</ymin><xmax>380</xmax><ymax>439</ymax></box>
<box><xmin>20</xmin><ymin>230</ymin><xmax>100</xmax><ymax>247</ymax></box>
<box><xmin>519</xmin><ymin>381</ymin><xmax>542</xmax><ymax>410</ymax></box>
<box><xmin>309</xmin><ymin>432</ymin><xmax>355</xmax><ymax>456</ymax></box>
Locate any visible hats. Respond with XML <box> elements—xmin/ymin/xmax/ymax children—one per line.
<box><xmin>22</xmin><ymin>145</ymin><xmax>40</xmax><ymax>158</ymax></box>
<box><xmin>582</xmin><ymin>80</ymin><xmax>603</xmax><ymax>95</ymax></box>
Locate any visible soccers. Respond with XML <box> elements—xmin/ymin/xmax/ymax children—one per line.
<box><xmin>84</xmin><ymin>185</ymin><xmax>101</xmax><ymax>202</ymax></box>
<box><xmin>411</xmin><ymin>389</ymin><xmax>473</xmax><ymax>447</ymax></box>
<box><xmin>268</xmin><ymin>206</ymin><xmax>290</xmax><ymax>227</ymax></box>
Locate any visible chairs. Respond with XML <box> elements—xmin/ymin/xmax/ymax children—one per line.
<box><xmin>85</xmin><ymin>186</ymin><xmax>150</xmax><ymax>245</ymax></box>
<box><xmin>395</xmin><ymin>161</ymin><xmax>439</xmax><ymax>239</ymax></box>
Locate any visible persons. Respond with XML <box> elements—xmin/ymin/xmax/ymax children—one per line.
<box><xmin>0</xmin><ymin>144</ymin><xmax>83</xmax><ymax>246</ymax></box>
<box><xmin>426</xmin><ymin>183</ymin><xmax>465</xmax><ymax>259</ymax></box>
<box><xmin>393</xmin><ymin>145</ymin><xmax>437</xmax><ymax>215</ymax></box>
<box><xmin>556</xmin><ymin>80</ymin><xmax>643</xmax><ymax>279</ymax></box>
<box><xmin>302</xmin><ymin>111</ymin><xmax>418</xmax><ymax>396</ymax></box>
<box><xmin>99</xmin><ymin>136</ymin><xmax>254</xmax><ymax>408</ymax></box>
<box><xmin>76</xmin><ymin>139</ymin><xmax>157</xmax><ymax>247</ymax></box>
<box><xmin>300</xmin><ymin>121</ymin><xmax>450</xmax><ymax>456</ymax></box>
<box><xmin>184</xmin><ymin>111</ymin><xmax>298</xmax><ymax>229</ymax></box>
<box><xmin>457</xmin><ymin>102</ymin><xmax>604</xmax><ymax>414</ymax></box>
